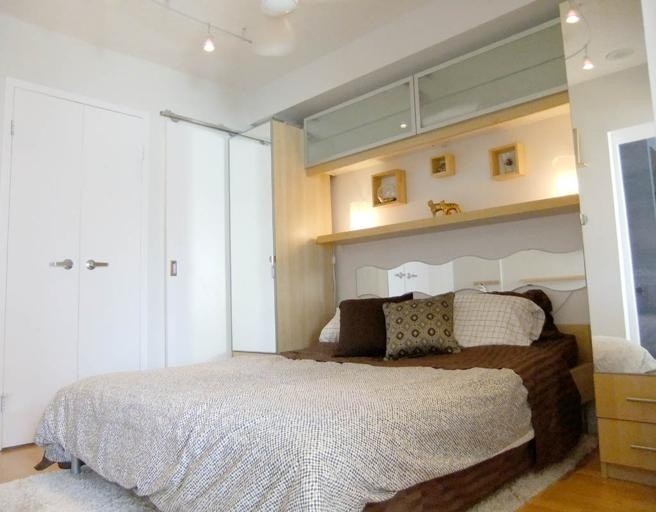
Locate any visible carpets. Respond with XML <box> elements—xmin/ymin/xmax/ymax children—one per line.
<box><xmin>0</xmin><ymin>431</ymin><xmax>598</xmax><ymax>512</ymax></box>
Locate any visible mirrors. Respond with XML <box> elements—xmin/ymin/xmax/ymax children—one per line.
<box><xmin>227</xmin><ymin>118</ymin><xmax>275</xmax><ymax>354</ymax></box>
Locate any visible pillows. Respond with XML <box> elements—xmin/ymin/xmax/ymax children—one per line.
<box><xmin>318</xmin><ymin>287</ymin><xmax>565</xmax><ymax>365</ymax></box>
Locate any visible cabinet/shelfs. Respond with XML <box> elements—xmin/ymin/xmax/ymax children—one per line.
<box><xmin>591</xmin><ymin>368</ymin><xmax>656</xmax><ymax>489</ymax></box>
<box><xmin>0</xmin><ymin>77</ymin><xmax>149</xmax><ymax>451</ymax></box>
<box><xmin>160</xmin><ymin>112</ymin><xmax>227</xmax><ymax>369</ymax></box>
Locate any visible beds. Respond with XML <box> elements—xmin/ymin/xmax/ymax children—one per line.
<box><xmin>33</xmin><ymin>323</ymin><xmax>593</xmax><ymax>512</ymax></box>
